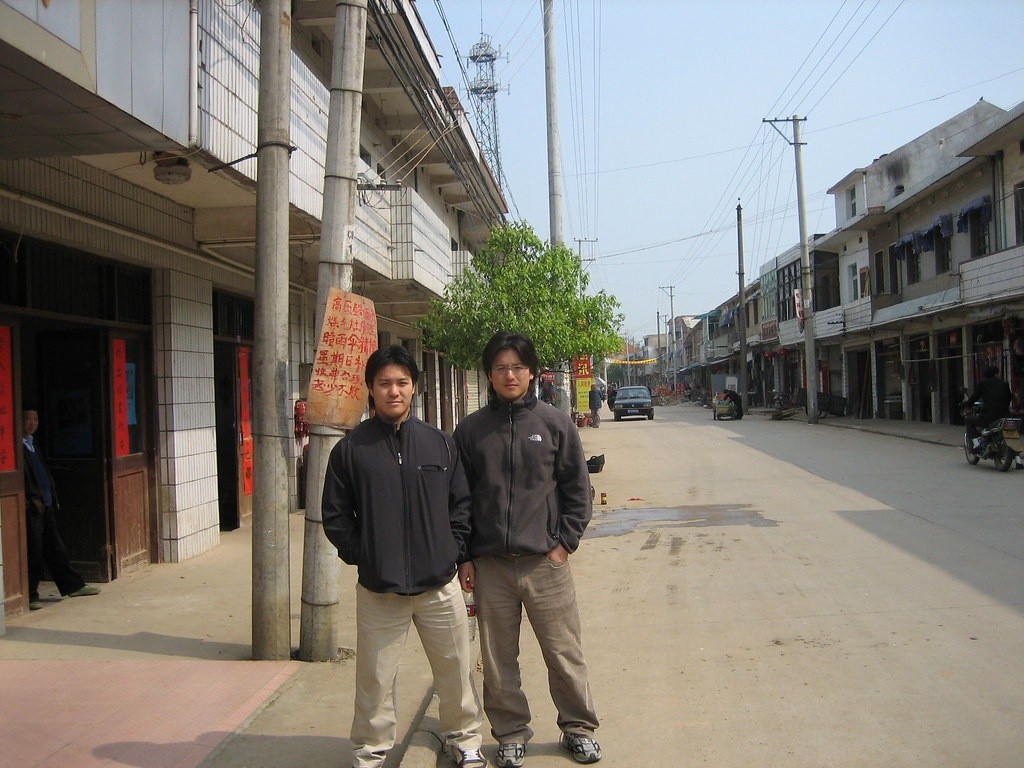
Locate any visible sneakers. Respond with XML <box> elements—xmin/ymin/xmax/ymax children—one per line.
<box><xmin>560</xmin><ymin>729</ymin><xmax>602</xmax><ymax>764</ymax></box>
<box><xmin>27</xmin><ymin>601</ymin><xmax>42</xmax><ymax>610</ymax></box>
<box><xmin>68</xmin><ymin>584</ymin><xmax>99</xmax><ymax>597</ymax></box>
<box><xmin>495</xmin><ymin>741</ymin><xmax>529</xmax><ymax>767</ymax></box>
<box><xmin>450</xmin><ymin>745</ymin><xmax>488</xmax><ymax>768</ymax></box>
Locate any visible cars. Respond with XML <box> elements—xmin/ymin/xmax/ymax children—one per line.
<box><xmin>609</xmin><ymin>384</ymin><xmax>654</xmax><ymax>425</ymax></box>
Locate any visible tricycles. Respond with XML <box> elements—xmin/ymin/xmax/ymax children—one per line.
<box><xmin>713</xmin><ymin>393</ymin><xmax>737</xmax><ymax>421</ymax></box>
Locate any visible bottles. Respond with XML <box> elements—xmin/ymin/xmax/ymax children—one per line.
<box><xmin>463</xmin><ymin>580</ymin><xmax>476</xmax><ymax>641</ymax></box>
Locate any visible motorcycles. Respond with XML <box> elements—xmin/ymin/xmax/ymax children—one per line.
<box><xmin>957</xmin><ymin>386</ymin><xmax>1014</xmax><ymax>470</ymax></box>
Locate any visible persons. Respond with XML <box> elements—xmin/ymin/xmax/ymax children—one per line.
<box><xmin>321</xmin><ymin>344</ymin><xmax>488</xmax><ymax>768</ymax></box>
<box><xmin>452</xmin><ymin>330</ymin><xmax>604</xmax><ymax>765</ymax></box>
<box><xmin>16</xmin><ymin>403</ymin><xmax>102</xmax><ymax>611</ymax></box>
<box><xmin>586</xmin><ymin>383</ymin><xmax>743</xmax><ymax>429</ymax></box>
<box><xmin>957</xmin><ymin>366</ymin><xmax>1011</xmax><ymax>454</ymax></box>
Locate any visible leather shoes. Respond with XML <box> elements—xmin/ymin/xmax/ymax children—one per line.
<box><xmin>968</xmin><ymin>445</ymin><xmax>981</xmax><ymax>452</ymax></box>
<box><xmin>1015</xmin><ymin>463</ymin><xmax>1024</xmax><ymax>469</ymax></box>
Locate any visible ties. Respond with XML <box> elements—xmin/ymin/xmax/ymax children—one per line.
<box><xmin>25</xmin><ymin>440</ymin><xmax>33</xmax><ymax>452</ymax></box>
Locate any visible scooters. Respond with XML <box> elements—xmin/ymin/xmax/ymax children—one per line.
<box><xmin>773</xmin><ymin>389</ymin><xmax>784</xmax><ymax>413</ymax></box>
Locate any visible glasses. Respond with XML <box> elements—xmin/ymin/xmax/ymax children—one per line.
<box><xmin>488</xmin><ymin>364</ymin><xmax>530</xmax><ymax>373</ymax></box>
